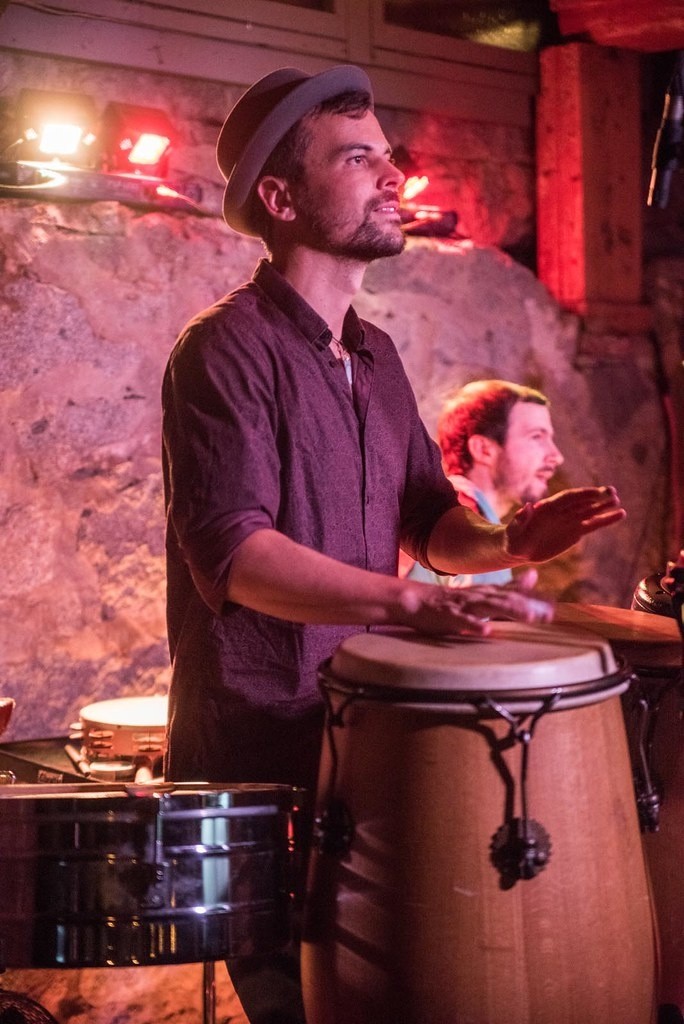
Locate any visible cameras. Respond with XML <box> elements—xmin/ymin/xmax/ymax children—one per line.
<box><xmin>645</xmin><ymin>56</ymin><xmax>684</xmax><ymax>210</ymax></box>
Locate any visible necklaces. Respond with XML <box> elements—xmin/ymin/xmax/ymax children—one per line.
<box><xmin>332</xmin><ymin>337</ymin><xmax>348</xmax><ymax>361</ymax></box>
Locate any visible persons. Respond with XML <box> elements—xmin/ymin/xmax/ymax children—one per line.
<box><xmin>395</xmin><ymin>379</ymin><xmax>563</xmax><ymax>622</ymax></box>
<box><xmin>663</xmin><ymin>551</ymin><xmax>684</xmax><ymax>628</ymax></box>
<box><xmin>162</xmin><ymin>63</ymin><xmax>626</xmax><ymax>1024</ymax></box>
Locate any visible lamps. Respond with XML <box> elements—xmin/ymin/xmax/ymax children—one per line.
<box><xmin>100</xmin><ymin>101</ymin><xmax>179</xmax><ymax>181</ymax></box>
<box><xmin>12</xmin><ymin>88</ymin><xmax>115</xmax><ymax>168</ymax></box>
<box><xmin>390</xmin><ymin>144</ymin><xmax>429</xmax><ymax>200</ymax></box>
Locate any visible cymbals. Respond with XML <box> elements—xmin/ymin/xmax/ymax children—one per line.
<box><xmin>69</xmin><ymin>722</ymin><xmax>164</xmax><ymax>752</ymax></box>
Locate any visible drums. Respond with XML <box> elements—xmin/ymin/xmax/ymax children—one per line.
<box><xmin>454</xmin><ymin>606</ymin><xmax>684</xmax><ymax>837</ymax></box>
<box><xmin>308</xmin><ymin>629</ymin><xmax>662</xmax><ymax>1024</ymax></box>
<box><xmin>0</xmin><ymin>785</ymin><xmax>309</xmax><ymax>971</ymax></box>
<box><xmin>79</xmin><ymin>695</ymin><xmax>170</xmax><ymax>773</ymax></box>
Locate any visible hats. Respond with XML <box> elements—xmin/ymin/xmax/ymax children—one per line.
<box><xmin>216</xmin><ymin>66</ymin><xmax>376</xmax><ymax>237</ymax></box>
